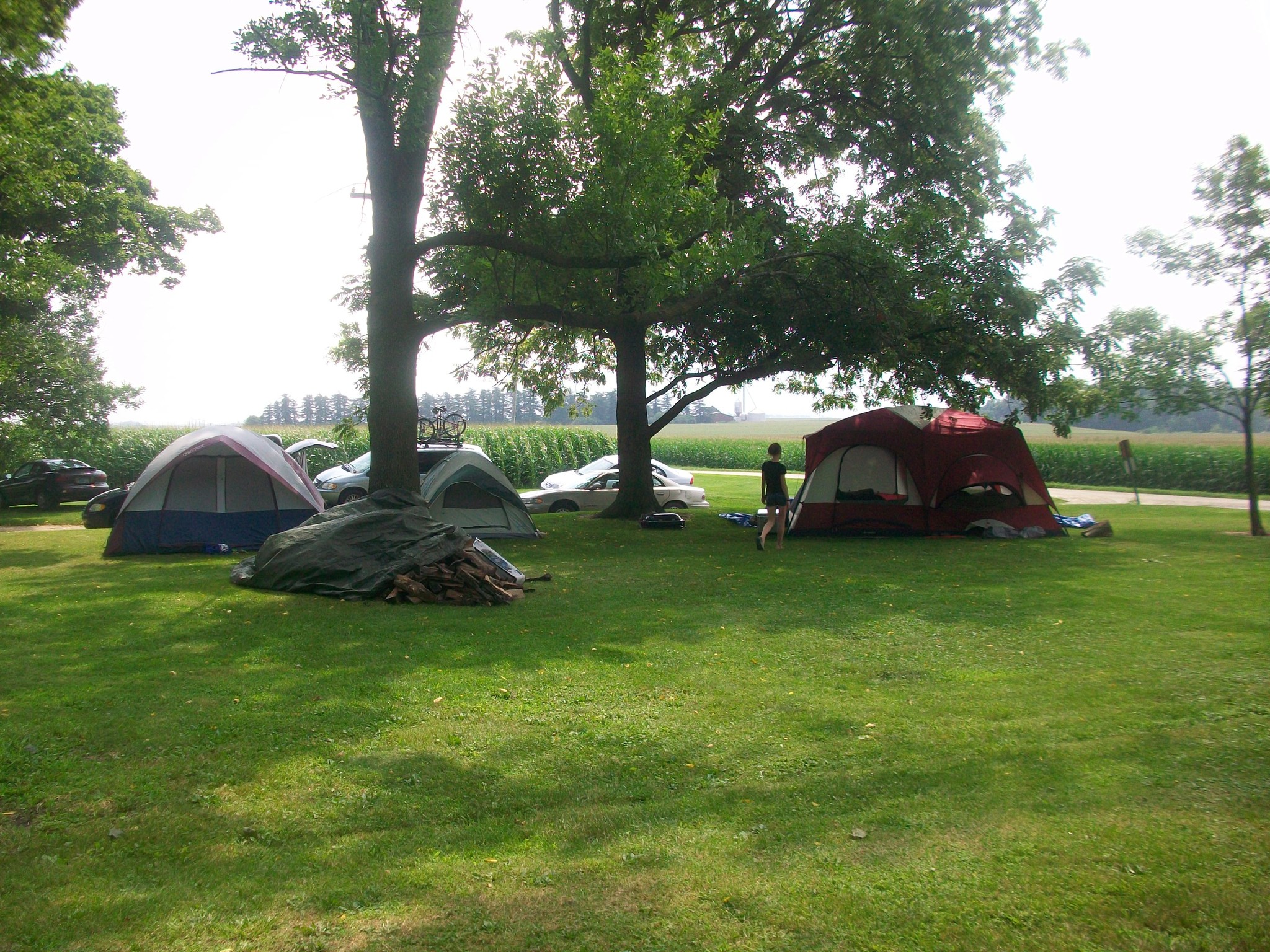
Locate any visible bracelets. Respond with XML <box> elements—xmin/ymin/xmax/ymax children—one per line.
<box><xmin>787</xmin><ymin>500</ymin><xmax>790</xmax><ymax>501</ymax></box>
<box><xmin>761</xmin><ymin>495</ymin><xmax>766</xmax><ymax>497</ymax></box>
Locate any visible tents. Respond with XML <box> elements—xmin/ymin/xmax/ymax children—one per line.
<box><xmin>787</xmin><ymin>405</ymin><xmax>1070</xmax><ymax>536</ymax></box>
<box><xmin>102</xmin><ymin>426</ymin><xmax>325</xmax><ymax>556</ymax></box>
<box><xmin>421</xmin><ymin>450</ymin><xmax>540</xmax><ymax>539</ymax></box>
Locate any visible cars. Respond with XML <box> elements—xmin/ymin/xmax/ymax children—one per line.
<box><xmin>518</xmin><ymin>468</ymin><xmax>710</xmax><ymax>514</ymax></box>
<box><xmin>539</xmin><ymin>455</ymin><xmax>694</xmax><ymax>487</ymax></box>
<box><xmin>0</xmin><ymin>458</ymin><xmax>109</xmax><ymax>511</ymax></box>
<box><xmin>80</xmin><ymin>434</ymin><xmax>338</xmax><ymax>530</ymax></box>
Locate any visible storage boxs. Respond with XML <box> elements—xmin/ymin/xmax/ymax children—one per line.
<box><xmin>756</xmin><ymin>509</ymin><xmax>779</xmax><ymax>533</ymax></box>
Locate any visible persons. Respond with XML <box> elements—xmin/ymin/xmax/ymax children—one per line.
<box><xmin>756</xmin><ymin>443</ymin><xmax>791</xmax><ymax>551</ymax></box>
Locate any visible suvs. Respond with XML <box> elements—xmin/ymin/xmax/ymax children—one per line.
<box><xmin>312</xmin><ymin>444</ymin><xmax>482</xmax><ymax>507</ymax></box>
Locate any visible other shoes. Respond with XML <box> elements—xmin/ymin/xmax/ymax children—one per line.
<box><xmin>756</xmin><ymin>537</ymin><xmax>764</xmax><ymax>550</ymax></box>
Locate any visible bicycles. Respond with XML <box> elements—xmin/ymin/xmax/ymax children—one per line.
<box><xmin>416</xmin><ymin>404</ymin><xmax>470</xmax><ymax>443</ymax></box>
